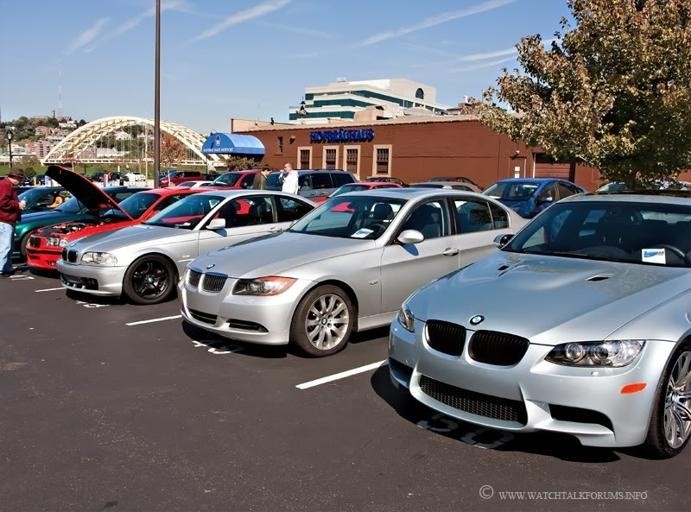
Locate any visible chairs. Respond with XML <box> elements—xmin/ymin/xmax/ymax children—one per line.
<box><xmin>366</xmin><ymin>204</ymin><xmax>394</xmax><ymax>239</ymax></box>
<box><xmin>219</xmin><ymin>205</ymin><xmax>265</xmax><ymax>228</ymax></box>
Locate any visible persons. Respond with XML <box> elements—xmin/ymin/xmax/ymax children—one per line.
<box><xmin>0</xmin><ymin>169</ymin><xmax>26</xmax><ymax>277</ymax></box>
<box><xmin>278</xmin><ymin>163</ymin><xmax>298</xmax><ymax>208</ymax></box>
<box><xmin>254</xmin><ymin>164</ymin><xmax>273</xmax><ymax>190</ymax></box>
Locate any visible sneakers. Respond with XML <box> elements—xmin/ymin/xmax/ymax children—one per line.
<box><xmin>0</xmin><ymin>270</ymin><xmax>22</xmax><ymax>277</ymax></box>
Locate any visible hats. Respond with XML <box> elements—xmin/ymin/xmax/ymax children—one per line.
<box><xmin>5</xmin><ymin>169</ymin><xmax>24</xmax><ymax>178</ymax></box>
